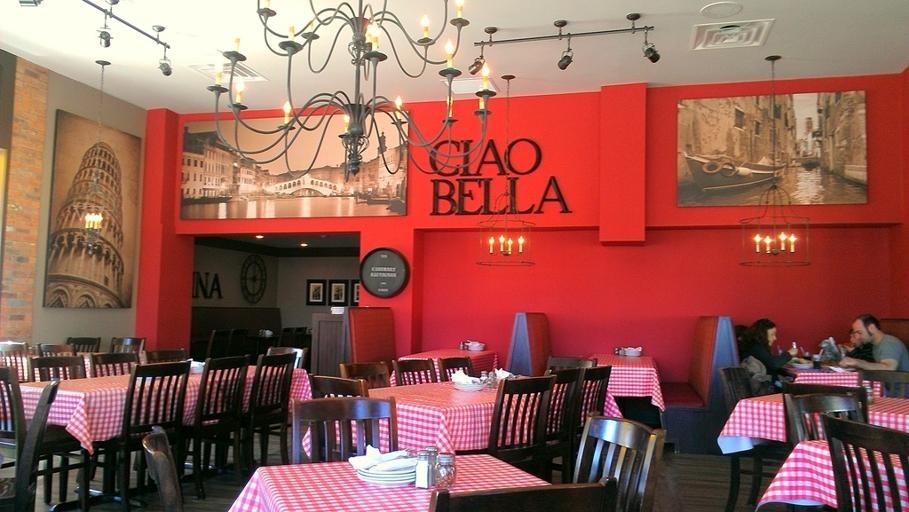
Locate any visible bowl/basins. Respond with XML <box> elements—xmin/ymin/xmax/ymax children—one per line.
<box><xmin>624</xmin><ymin>348</ymin><xmax>641</xmax><ymax>357</ymax></box>
<box><xmin>331</xmin><ymin>307</ymin><xmax>345</xmax><ymax>314</ymax></box>
<box><xmin>189</xmin><ymin>360</ymin><xmax>204</xmax><ymax>374</ymax></box>
<box><xmin>468</xmin><ymin>342</ymin><xmax>485</xmax><ymax>351</ymax></box>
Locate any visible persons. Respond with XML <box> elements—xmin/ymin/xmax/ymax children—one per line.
<box><xmin>736</xmin><ymin>325</ymin><xmax>748</xmax><ymax>346</ymax></box>
<box><xmin>844</xmin><ymin>329</ymin><xmax>874</xmax><ymax>361</ymax></box>
<box><xmin>837</xmin><ymin>312</ymin><xmax>909</xmax><ymax>399</ymax></box>
<box><xmin>741</xmin><ymin>319</ymin><xmax>799</xmax><ymax>394</ymax></box>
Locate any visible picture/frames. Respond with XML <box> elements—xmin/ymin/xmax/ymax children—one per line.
<box><xmin>306</xmin><ymin>278</ymin><xmax>327</xmax><ymax>305</ymax></box>
<box><xmin>328</xmin><ymin>279</ymin><xmax>349</xmax><ymax>304</ymax></box>
<box><xmin>350</xmin><ymin>279</ymin><xmax>359</xmax><ymax>305</ymax></box>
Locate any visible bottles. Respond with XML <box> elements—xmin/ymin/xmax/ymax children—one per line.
<box><xmin>460</xmin><ymin>341</ymin><xmax>469</xmax><ymax>350</ymax></box>
<box><xmin>481</xmin><ymin>370</ymin><xmax>498</xmax><ymax>388</ymax></box>
<box><xmin>614</xmin><ymin>346</ymin><xmax>624</xmax><ymax>356</ymax></box>
<box><xmin>412</xmin><ymin>446</ymin><xmax>456</xmax><ymax>490</ymax></box>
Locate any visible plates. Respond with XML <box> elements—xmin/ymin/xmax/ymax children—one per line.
<box><xmin>788</xmin><ymin>342</ymin><xmax>854</xmax><ymax>369</ymax></box>
<box><xmin>357</xmin><ymin>456</ymin><xmax>418</xmax><ymax>489</ymax></box>
<box><xmin>454</xmin><ymin>377</ymin><xmax>481</xmax><ymax>392</ymax></box>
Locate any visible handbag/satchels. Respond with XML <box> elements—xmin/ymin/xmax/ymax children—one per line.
<box><xmin>741</xmin><ymin>356</ymin><xmax>772</xmax><ymax>396</ymax></box>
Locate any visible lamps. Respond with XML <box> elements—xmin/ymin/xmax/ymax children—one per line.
<box><xmin>83</xmin><ymin>60</ymin><xmax>112</xmax><ymax>250</ymax></box>
<box><xmin>19</xmin><ymin>0</ymin><xmax>172</xmax><ymax>78</ymax></box>
<box><xmin>739</xmin><ymin>55</ymin><xmax>813</xmax><ymax>270</ymax></box>
<box><xmin>479</xmin><ymin>74</ymin><xmax>535</xmax><ymax>273</ymax></box>
<box><xmin>204</xmin><ymin>1</ymin><xmax>510</xmax><ymax>186</ymax></box>
<box><xmin>467</xmin><ymin>14</ymin><xmax>661</xmax><ymax>75</ymax></box>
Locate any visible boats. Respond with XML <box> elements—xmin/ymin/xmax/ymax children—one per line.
<box><xmin>680</xmin><ymin>141</ymin><xmax>801</xmax><ymax>192</ymax></box>
<box><xmin>327</xmin><ymin>189</ymin><xmax>403</xmax><ymax>213</ymax></box>
<box><xmin>183</xmin><ymin>193</ymin><xmax>234</xmax><ymax>207</ymax></box>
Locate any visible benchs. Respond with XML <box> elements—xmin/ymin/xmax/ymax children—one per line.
<box><xmin>340</xmin><ymin>306</ymin><xmax>396</xmax><ymax>389</ymax></box>
<box><xmin>658</xmin><ymin>314</ymin><xmax>737</xmax><ymax>455</ymax></box>
